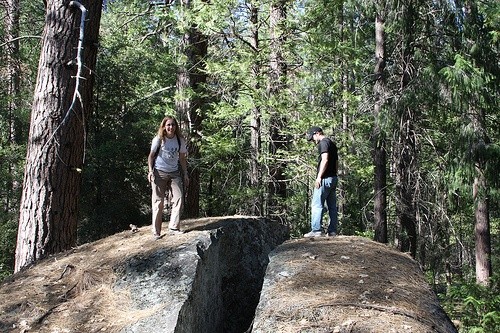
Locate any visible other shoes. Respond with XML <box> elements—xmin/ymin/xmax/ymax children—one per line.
<box><xmin>154</xmin><ymin>233</ymin><xmax>160</xmax><ymax>239</ymax></box>
<box><xmin>169</xmin><ymin>229</ymin><xmax>184</xmax><ymax>235</ymax></box>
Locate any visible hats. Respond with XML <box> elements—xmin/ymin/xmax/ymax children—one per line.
<box><xmin>307</xmin><ymin>127</ymin><xmax>322</xmax><ymax>141</ymax></box>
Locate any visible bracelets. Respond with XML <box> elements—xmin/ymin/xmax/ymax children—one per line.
<box><xmin>148</xmin><ymin>170</ymin><xmax>153</xmax><ymax>172</ymax></box>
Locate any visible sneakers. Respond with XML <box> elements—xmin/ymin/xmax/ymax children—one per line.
<box><xmin>304</xmin><ymin>231</ymin><xmax>325</xmax><ymax>237</ymax></box>
<box><xmin>327</xmin><ymin>232</ymin><xmax>336</xmax><ymax>237</ymax></box>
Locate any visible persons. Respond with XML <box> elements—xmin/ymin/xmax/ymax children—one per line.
<box><xmin>147</xmin><ymin>116</ymin><xmax>190</xmax><ymax>239</ymax></box>
<box><xmin>304</xmin><ymin>127</ymin><xmax>339</xmax><ymax>239</ymax></box>
